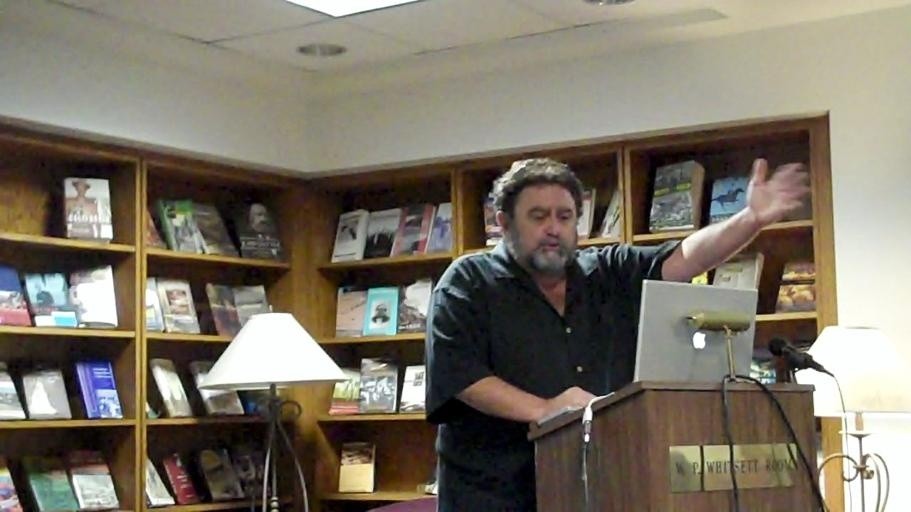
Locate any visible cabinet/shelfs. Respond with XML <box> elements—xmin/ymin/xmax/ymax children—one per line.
<box><xmin>0</xmin><ymin>117</ymin><xmax>841</xmax><ymax>512</ymax></box>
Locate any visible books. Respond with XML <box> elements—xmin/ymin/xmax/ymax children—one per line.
<box><xmin>690</xmin><ymin>252</ymin><xmax>816</xmax><ymax>314</ymax></box>
<box><xmin>20</xmin><ymin>361</ymin><xmax>72</xmax><ymax>421</ymax></box>
<box><xmin>146</xmin><ymin>198</ymin><xmax>241</xmax><ymax>258</ymax></box>
<box><xmin>145</xmin><ymin>277</ymin><xmax>270</xmax><ymax>336</ymax></box>
<box><xmin>648</xmin><ymin>161</ymin><xmax>752</xmax><ymax>234</ymax></box>
<box><xmin>75</xmin><ymin>359</ymin><xmax>124</xmax><ymax>419</ymax></box>
<box><xmin>0</xmin><ymin>360</ymin><xmax>28</xmax><ymax>421</ymax></box>
<box><xmin>749</xmin><ymin>340</ymin><xmax>811</xmax><ymax>384</ymax></box>
<box><xmin>1</xmin><ymin>260</ymin><xmax>120</xmax><ymax>330</ymax></box>
<box><xmin>233</xmin><ymin>202</ymin><xmax>289</xmax><ymax>262</ymax></box>
<box><xmin>329</xmin><ymin>202</ymin><xmax>453</xmax><ymax>264</ymax></box>
<box><xmin>334</xmin><ymin>277</ymin><xmax>431</xmax><ymax>337</ymax></box>
<box><xmin>63</xmin><ymin>180</ymin><xmax>114</xmax><ymax>243</ymax></box>
<box><xmin>145</xmin><ymin>358</ymin><xmax>246</xmax><ymax>419</ymax></box>
<box><xmin>338</xmin><ymin>443</ymin><xmax>374</xmax><ymax>493</ymax></box>
<box><xmin>484</xmin><ymin>194</ymin><xmax>503</xmax><ymax>246</ymax></box>
<box><xmin>145</xmin><ymin>446</ymin><xmax>272</xmax><ymax>508</ymax></box>
<box><xmin>328</xmin><ymin>356</ymin><xmax>427</xmax><ymax>414</ymax></box>
<box><xmin>1</xmin><ymin>450</ymin><xmax>121</xmax><ymax>511</ymax></box>
<box><xmin>578</xmin><ymin>186</ymin><xmax>621</xmax><ymax>238</ymax></box>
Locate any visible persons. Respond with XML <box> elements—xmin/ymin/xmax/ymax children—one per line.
<box><xmin>64</xmin><ymin>180</ymin><xmax>102</xmax><ymax>239</ymax></box>
<box><xmin>245</xmin><ymin>203</ymin><xmax>270</xmax><ymax>235</ymax></box>
<box><xmin>425</xmin><ymin>157</ymin><xmax>813</xmax><ymax>512</ymax></box>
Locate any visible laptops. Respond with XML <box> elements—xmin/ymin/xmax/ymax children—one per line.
<box><xmin>632</xmin><ymin>279</ymin><xmax>759</xmax><ymax>382</ymax></box>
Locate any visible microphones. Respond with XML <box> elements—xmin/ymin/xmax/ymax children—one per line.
<box><xmin>768</xmin><ymin>336</ymin><xmax>823</xmax><ymax>372</ymax></box>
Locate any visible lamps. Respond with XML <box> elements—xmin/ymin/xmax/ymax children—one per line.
<box><xmin>794</xmin><ymin>325</ymin><xmax>911</xmax><ymax>512</ymax></box>
